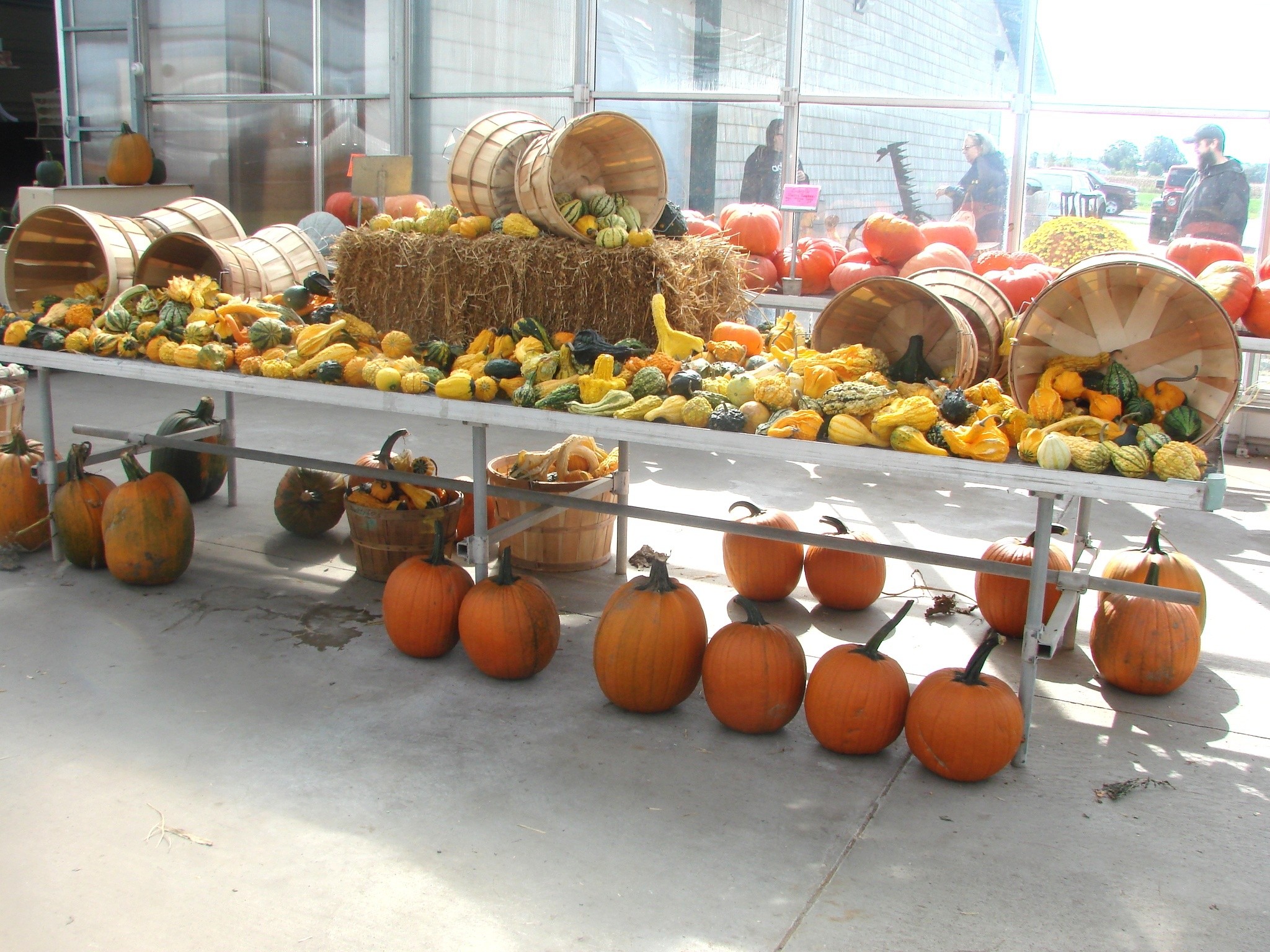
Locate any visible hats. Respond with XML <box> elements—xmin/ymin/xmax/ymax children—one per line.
<box><xmin>1182</xmin><ymin>124</ymin><xmax>1225</xmax><ymax>143</ymax></box>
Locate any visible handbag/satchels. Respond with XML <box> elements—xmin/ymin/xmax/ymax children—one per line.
<box><xmin>949</xmin><ymin>192</ymin><xmax>976</xmax><ymax>230</ymax></box>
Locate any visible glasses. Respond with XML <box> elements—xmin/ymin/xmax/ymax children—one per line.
<box><xmin>962</xmin><ymin>144</ymin><xmax>978</xmax><ymax>150</ymax></box>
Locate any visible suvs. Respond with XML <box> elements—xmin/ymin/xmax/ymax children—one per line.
<box><xmin>1147</xmin><ymin>164</ymin><xmax>1199</xmax><ymax>244</ymax></box>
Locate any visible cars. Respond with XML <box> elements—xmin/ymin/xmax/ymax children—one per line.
<box><xmin>1058</xmin><ymin>168</ymin><xmax>1139</xmax><ymax>217</ymax></box>
<box><xmin>1024</xmin><ymin>168</ymin><xmax>1106</xmax><ymax>238</ymax></box>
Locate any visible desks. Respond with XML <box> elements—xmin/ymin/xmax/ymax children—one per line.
<box><xmin>0</xmin><ymin>340</ymin><xmax>1224</xmax><ymax>769</ymax></box>
<box><xmin>18</xmin><ymin>184</ymin><xmax>198</xmax><ymax>223</ymax></box>
<box><xmin>743</xmin><ymin>289</ymin><xmax>1270</xmax><ymax>469</ymax></box>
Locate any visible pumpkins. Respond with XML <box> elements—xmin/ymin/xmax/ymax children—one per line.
<box><xmin>0</xmin><ymin>122</ymin><xmax>1270</xmax><ymax>782</ymax></box>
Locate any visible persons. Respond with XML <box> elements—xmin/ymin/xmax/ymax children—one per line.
<box><xmin>1166</xmin><ymin>124</ymin><xmax>1251</xmax><ymax>247</ymax></box>
<box><xmin>934</xmin><ymin>133</ymin><xmax>1008</xmax><ymax>253</ymax></box>
<box><xmin>736</xmin><ymin>118</ymin><xmax>810</xmax><ymax>209</ymax></box>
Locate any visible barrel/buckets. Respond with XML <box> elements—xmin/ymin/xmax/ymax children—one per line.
<box><xmin>450</xmin><ymin>106</ymin><xmax>670</xmax><ymax>241</ymax></box>
<box><xmin>4</xmin><ymin>192</ymin><xmax>331</xmax><ymax>318</ymax></box>
<box><xmin>808</xmin><ymin>244</ymin><xmax>1246</xmax><ymax>456</ymax></box>
<box><xmin>344</xmin><ymin>484</ymin><xmax>464</xmax><ymax>583</ymax></box>
<box><xmin>488</xmin><ymin>450</ymin><xmax>619</xmax><ymax>571</ymax></box>
